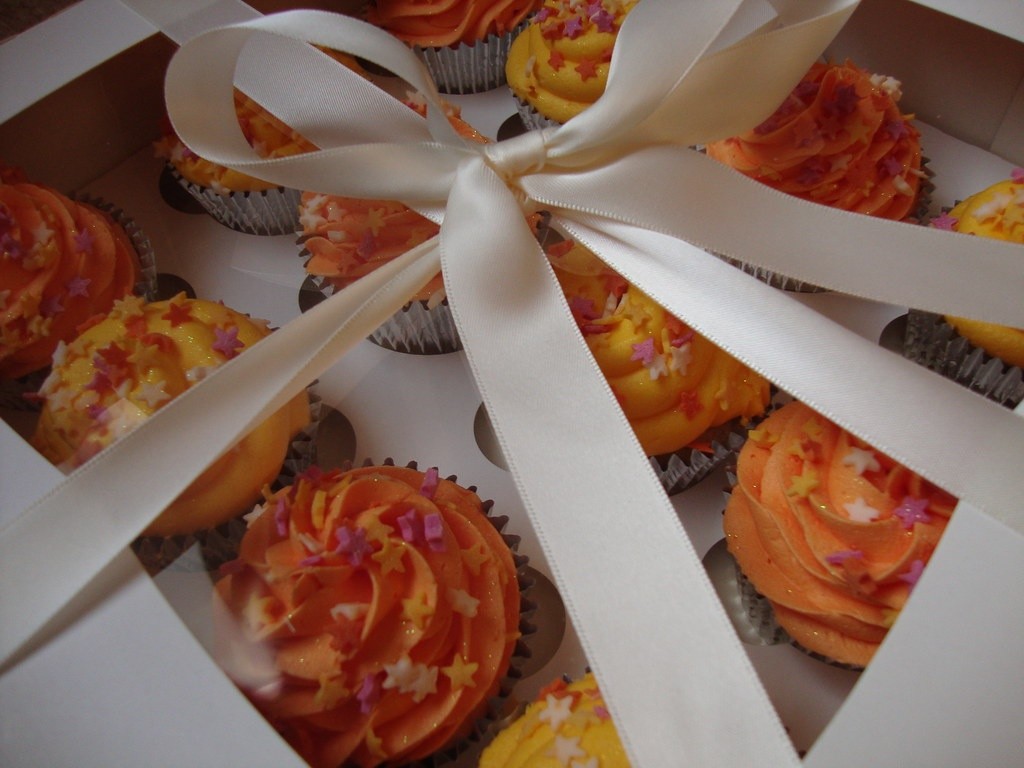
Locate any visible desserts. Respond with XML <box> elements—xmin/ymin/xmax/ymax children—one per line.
<box><xmin>0</xmin><ymin>0</ymin><xmax>1024</xmax><ymax>768</ymax></box>
